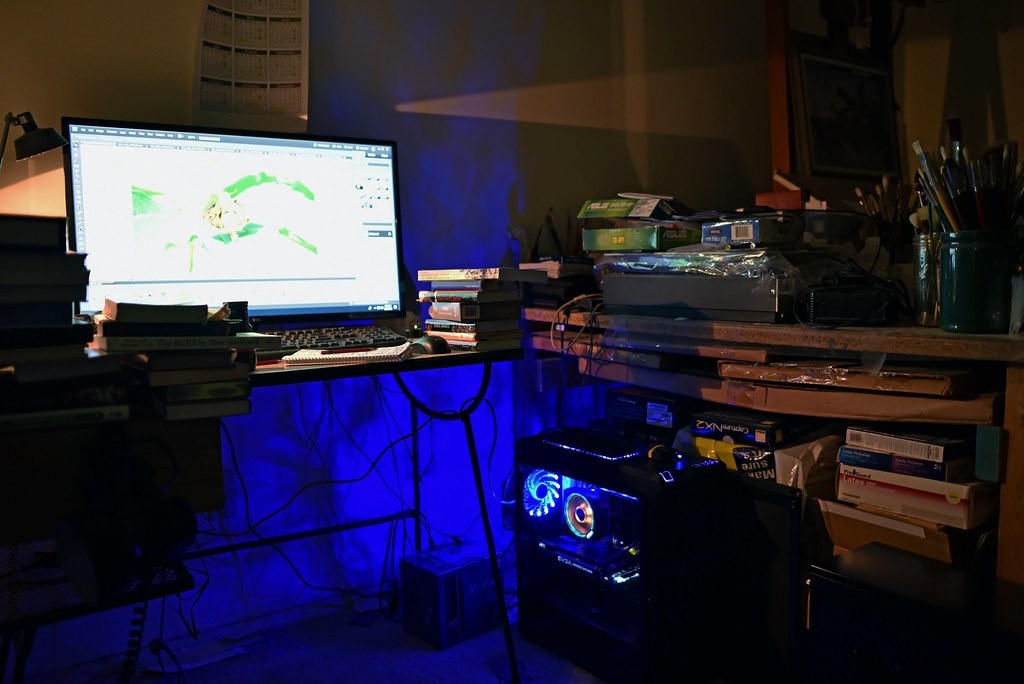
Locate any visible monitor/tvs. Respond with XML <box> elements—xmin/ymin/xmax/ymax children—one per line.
<box><xmin>62</xmin><ymin>118</ymin><xmax>408</xmax><ymax>326</ymax></box>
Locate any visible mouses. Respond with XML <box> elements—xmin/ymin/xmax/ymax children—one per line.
<box><xmin>409</xmin><ymin>336</ymin><xmax>452</xmax><ymax>354</ymax></box>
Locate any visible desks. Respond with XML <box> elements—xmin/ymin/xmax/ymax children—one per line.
<box><xmin>0</xmin><ymin>339</ymin><xmax>523</xmax><ymax>684</ymax></box>
<box><xmin>525</xmin><ymin>307</ymin><xmax>1024</xmax><ymax>584</ymax></box>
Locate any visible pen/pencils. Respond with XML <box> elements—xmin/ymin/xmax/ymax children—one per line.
<box><xmin>545</xmin><ymin>215</ymin><xmax>566</xmax><ymax>259</ymax></box>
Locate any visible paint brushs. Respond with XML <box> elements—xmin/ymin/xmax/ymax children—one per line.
<box><xmin>855</xmin><ymin>120</ymin><xmax>1024</xmax><ymax>231</ymax></box>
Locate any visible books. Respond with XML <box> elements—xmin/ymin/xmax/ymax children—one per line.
<box><xmin>0</xmin><ymin>214</ymin><xmax>282</xmax><ymax>420</ymax></box>
<box><xmin>282</xmin><ymin>341</ymin><xmax>412</xmax><ymax>365</ymax></box>
<box><xmin>417</xmin><ymin>267</ymin><xmax>548</xmax><ymax>351</ymax></box>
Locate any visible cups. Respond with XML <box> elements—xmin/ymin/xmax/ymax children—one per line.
<box><xmin>913</xmin><ymin>235</ymin><xmax>941</xmax><ymax>326</ymax></box>
<box><xmin>940</xmin><ymin>232</ymin><xmax>1011</xmax><ymax>332</ymax></box>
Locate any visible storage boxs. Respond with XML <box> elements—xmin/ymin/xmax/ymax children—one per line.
<box><xmin>701</xmin><ymin>219</ymin><xmax>803</xmax><ymax>250</ymax></box>
<box><xmin>836</xmin><ymin>464</ymin><xmax>992</xmax><ymax>530</ymax></box>
<box><xmin>689</xmin><ymin>407</ymin><xmax>842</xmax><ymax>483</ymax></box>
<box><xmin>582</xmin><ymin>225</ymin><xmax>700</xmax><ymax>254</ymax></box>
<box><xmin>775</xmin><ymin>436</ymin><xmax>844</xmax><ymax>521</ymax></box>
<box><xmin>400</xmin><ymin>549</ymin><xmax>507</xmax><ymax>650</ymax></box>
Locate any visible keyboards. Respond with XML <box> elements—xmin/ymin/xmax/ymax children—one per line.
<box><xmin>256</xmin><ymin>326</ymin><xmax>409</xmax><ymax>362</ymax></box>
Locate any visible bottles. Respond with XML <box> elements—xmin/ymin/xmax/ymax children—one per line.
<box><xmin>864</xmin><ymin>237</ymin><xmax>890</xmax><ymax>279</ymax></box>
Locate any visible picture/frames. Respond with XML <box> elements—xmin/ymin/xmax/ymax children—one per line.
<box><xmin>785</xmin><ymin>27</ymin><xmax>900</xmax><ymax>187</ymax></box>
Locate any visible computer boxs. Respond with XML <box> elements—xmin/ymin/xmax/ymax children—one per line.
<box><xmin>511</xmin><ymin>428</ymin><xmax>728</xmax><ymax>684</ymax></box>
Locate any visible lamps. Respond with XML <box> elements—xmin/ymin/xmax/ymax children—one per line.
<box><xmin>0</xmin><ymin>111</ymin><xmax>67</xmax><ymax>170</ymax></box>
